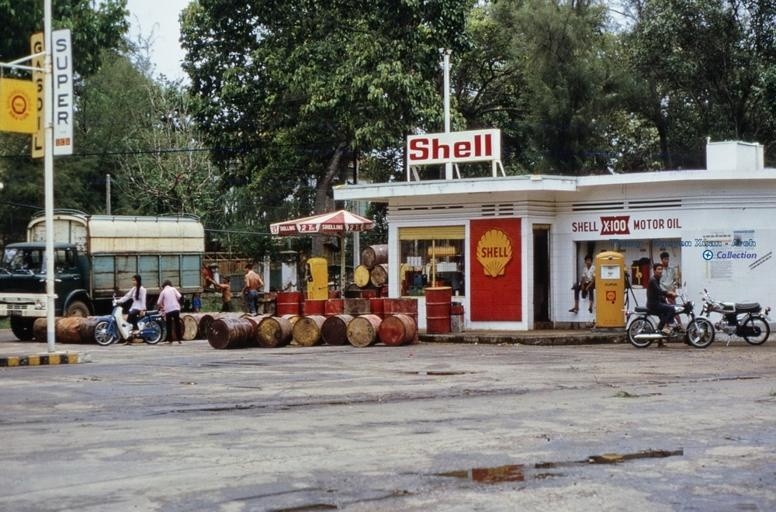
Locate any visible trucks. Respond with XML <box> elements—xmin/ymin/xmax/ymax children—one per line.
<box><xmin>0</xmin><ymin>206</ymin><xmax>206</xmax><ymax>342</ymax></box>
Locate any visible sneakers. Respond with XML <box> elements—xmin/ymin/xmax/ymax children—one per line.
<box><xmin>568</xmin><ymin>308</ymin><xmax>592</xmax><ymax>314</ymax></box>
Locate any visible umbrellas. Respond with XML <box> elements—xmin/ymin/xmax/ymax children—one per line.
<box><xmin>269</xmin><ymin>208</ymin><xmax>376</xmax><ymax>300</ymax></box>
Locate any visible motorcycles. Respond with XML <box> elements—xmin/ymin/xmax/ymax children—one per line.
<box><xmin>620</xmin><ymin>281</ymin><xmax>714</xmax><ymax>347</ymax></box>
<box><xmin>696</xmin><ymin>288</ymin><xmax>773</xmax><ymax>347</ymax></box>
<box><xmin>94</xmin><ymin>292</ymin><xmax>166</xmax><ymax>344</ymax></box>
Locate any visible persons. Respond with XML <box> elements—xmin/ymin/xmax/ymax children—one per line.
<box><xmin>244</xmin><ymin>264</ymin><xmax>264</xmax><ymax>313</ymax></box>
<box><xmin>567</xmin><ymin>254</ymin><xmax>595</xmax><ymax>314</ymax></box>
<box><xmin>156</xmin><ymin>279</ymin><xmax>183</xmax><ymax>345</ymax></box>
<box><xmin>658</xmin><ymin>252</ymin><xmax>683</xmax><ymax>329</ymax></box>
<box><xmin>205</xmin><ymin>275</ymin><xmax>236</xmax><ymax>313</ymax></box>
<box><xmin>645</xmin><ymin>262</ymin><xmax>679</xmax><ymax>348</ymax></box>
<box><xmin>116</xmin><ymin>274</ymin><xmax>147</xmax><ymax>346</ymax></box>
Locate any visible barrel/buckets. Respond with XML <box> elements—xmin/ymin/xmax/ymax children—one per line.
<box><xmin>425</xmin><ymin>286</ymin><xmax>452</xmax><ymax>333</ymax></box>
<box><xmin>347</xmin><ymin>310</ymin><xmax>380</xmax><ymax>347</ymax></box>
<box><xmin>304</xmin><ymin>297</ymin><xmax>418</xmax><ymax>313</ymax></box>
<box><xmin>354</xmin><ymin>244</ymin><xmax>388</xmax><ymax>287</ymax></box>
<box><xmin>160</xmin><ymin>309</ymin><xmax>213</xmax><ymax>342</ymax></box>
<box><xmin>255</xmin><ymin>314</ymin><xmax>291</xmax><ymax>347</ymax></box>
<box><xmin>377</xmin><ymin>313</ymin><xmax>417</xmax><ymax>343</ymax></box>
<box><xmin>321</xmin><ymin>312</ymin><xmax>353</xmax><ymax>343</ymax></box>
<box><xmin>276</xmin><ymin>291</ymin><xmax>301</xmax><ymax>313</ymax></box>
<box><xmin>293</xmin><ymin>313</ymin><xmax>324</xmax><ymax>346</ymax></box>
<box><xmin>209</xmin><ymin>316</ymin><xmax>252</xmax><ymax>349</ymax></box>
<box><xmin>33</xmin><ymin>311</ymin><xmax>122</xmax><ymax>345</ymax></box>
